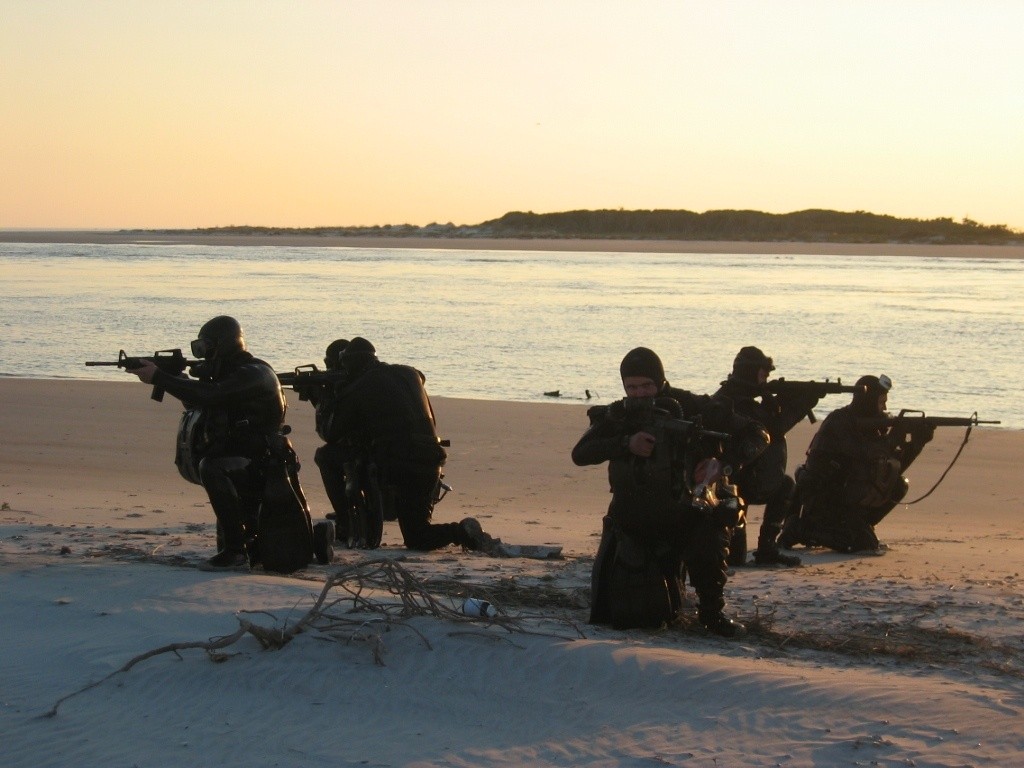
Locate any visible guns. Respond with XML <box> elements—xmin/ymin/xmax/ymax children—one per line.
<box><xmin>85</xmin><ymin>348</ymin><xmax>205</xmax><ymax>403</ymax></box>
<box><xmin>757</xmin><ymin>377</ymin><xmax>857</xmax><ymax>425</ymax></box>
<box><xmin>273</xmin><ymin>364</ymin><xmax>336</xmax><ymax>402</ymax></box>
<box><xmin>879</xmin><ymin>410</ymin><xmax>1002</xmax><ymax>505</ymax></box>
<box><xmin>607</xmin><ymin>393</ymin><xmax>733</xmax><ymax>471</ymax></box>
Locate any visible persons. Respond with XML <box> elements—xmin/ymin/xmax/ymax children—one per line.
<box><xmin>571</xmin><ymin>347</ymin><xmax>772</xmax><ymax>639</ymax></box>
<box><xmin>132</xmin><ymin>315</ymin><xmax>336</xmax><ymax>571</ymax></box>
<box><xmin>314</xmin><ymin>336</ymin><xmax>493</xmax><ymax>551</ymax></box>
<box><xmin>776</xmin><ymin>374</ymin><xmax>937</xmax><ymax>556</ymax></box>
<box><xmin>711</xmin><ymin>346</ymin><xmax>827</xmax><ymax>566</ymax></box>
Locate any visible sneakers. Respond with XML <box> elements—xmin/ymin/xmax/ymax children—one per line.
<box><xmin>756</xmin><ymin>537</ymin><xmax>802</xmax><ymax>565</ymax></box>
<box><xmin>458</xmin><ymin>517</ymin><xmax>504</xmax><ymax>558</ymax></box>
<box><xmin>697</xmin><ymin>603</ymin><xmax>745</xmax><ymax>639</ymax></box>
<box><xmin>778</xmin><ymin>514</ymin><xmax>800</xmax><ymax>548</ymax></box>
<box><xmin>314</xmin><ymin>521</ymin><xmax>336</xmax><ymax>564</ymax></box>
<box><xmin>199</xmin><ymin>549</ymin><xmax>248</xmax><ymax>572</ymax></box>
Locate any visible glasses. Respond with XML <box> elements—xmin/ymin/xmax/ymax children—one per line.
<box><xmin>191</xmin><ymin>338</ymin><xmax>211</xmax><ymax>358</ymax></box>
